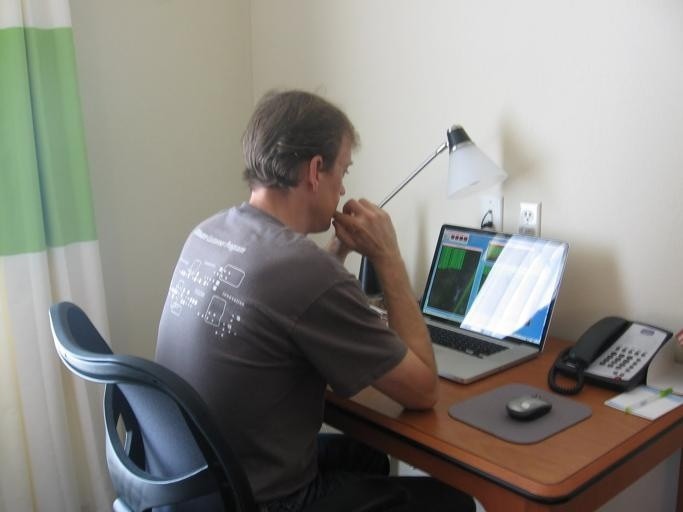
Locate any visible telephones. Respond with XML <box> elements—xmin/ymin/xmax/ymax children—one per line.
<box><xmin>555</xmin><ymin>317</ymin><xmax>674</xmax><ymax>390</ymax></box>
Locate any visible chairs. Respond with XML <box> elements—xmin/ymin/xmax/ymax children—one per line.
<box><xmin>48</xmin><ymin>301</ymin><xmax>260</xmax><ymax>511</ymax></box>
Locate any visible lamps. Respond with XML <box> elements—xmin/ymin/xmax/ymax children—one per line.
<box><xmin>354</xmin><ymin>125</ymin><xmax>507</xmax><ymax>306</ymax></box>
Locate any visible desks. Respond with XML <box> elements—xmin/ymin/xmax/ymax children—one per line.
<box><xmin>321</xmin><ymin>333</ymin><xmax>683</xmax><ymax>511</ymax></box>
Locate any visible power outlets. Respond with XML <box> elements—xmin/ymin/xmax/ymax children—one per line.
<box><xmin>517</xmin><ymin>202</ymin><xmax>540</xmax><ymax>239</ymax></box>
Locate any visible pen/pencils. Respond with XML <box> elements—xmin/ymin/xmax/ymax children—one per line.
<box><xmin>625</xmin><ymin>388</ymin><xmax>673</xmax><ymax>411</ymax></box>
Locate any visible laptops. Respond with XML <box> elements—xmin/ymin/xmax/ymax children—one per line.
<box><xmin>419</xmin><ymin>222</ymin><xmax>569</xmax><ymax>385</ymax></box>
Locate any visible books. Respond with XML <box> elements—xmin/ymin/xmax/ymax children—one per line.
<box><xmin>603</xmin><ymin>383</ymin><xmax>683</xmax><ymax>421</ymax></box>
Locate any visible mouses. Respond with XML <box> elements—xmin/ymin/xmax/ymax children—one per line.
<box><xmin>506</xmin><ymin>396</ymin><xmax>553</xmax><ymax>421</ymax></box>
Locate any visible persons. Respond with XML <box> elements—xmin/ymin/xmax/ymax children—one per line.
<box><xmin>147</xmin><ymin>87</ymin><xmax>477</xmax><ymax>512</ymax></box>
